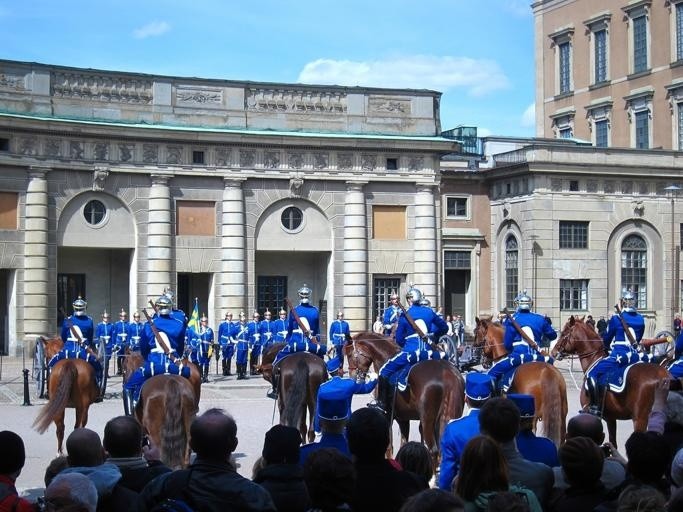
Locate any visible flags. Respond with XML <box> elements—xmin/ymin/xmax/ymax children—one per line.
<box><xmin>187</xmin><ymin>303</ymin><xmax>202</xmax><ymax>353</ymax></box>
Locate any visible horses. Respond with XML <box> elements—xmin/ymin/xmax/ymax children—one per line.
<box><xmin>173</xmin><ymin>356</ymin><xmax>202</xmax><ymax>407</ymax></box>
<box><xmin>550</xmin><ymin>315</ymin><xmax>675</xmax><ymax>452</ymax></box>
<box><xmin>473</xmin><ymin>314</ymin><xmax>569</xmax><ymax>449</ymax></box>
<box><xmin>120</xmin><ymin>349</ymin><xmax>200</xmax><ymax>470</ymax></box>
<box><xmin>342</xmin><ymin>330</ymin><xmax>466</xmax><ymax>486</ymax></box>
<box><xmin>252</xmin><ymin>341</ymin><xmax>328</xmax><ymax>445</ymax></box>
<box><xmin>29</xmin><ymin>334</ymin><xmax>97</xmax><ymax>459</ymax></box>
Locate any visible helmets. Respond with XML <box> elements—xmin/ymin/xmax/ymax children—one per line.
<box><xmin>621</xmin><ymin>288</ymin><xmax>636</xmax><ymax>307</ymax></box>
<box><xmin>298</xmin><ymin>283</ymin><xmax>312</xmax><ymax>304</ymax></box>
<box><xmin>337</xmin><ymin>309</ymin><xmax>343</xmax><ymax>319</ymax></box>
<box><xmin>390</xmin><ymin>289</ymin><xmax>399</xmax><ymax>299</ymax></box>
<box><xmin>102</xmin><ymin>308</ymin><xmax>140</xmax><ymax>321</ymax></box>
<box><xmin>407</xmin><ymin>280</ymin><xmax>431</xmax><ymax>308</ymax></box>
<box><xmin>201</xmin><ymin>312</ymin><xmax>208</xmax><ymax>323</ymax></box>
<box><xmin>73</xmin><ymin>296</ymin><xmax>88</xmax><ymax>316</ymax></box>
<box><xmin>518</xmin><ymin>289</ymin><xmax>532</xmax><ymax>310</ymax></box>
<box><xmin>225</xmin><ymin>306</ymin><xmax>286</xmax><ymax>321</ymax></box>
<box><xmin>155</xmin><ymin>286</ymin><xmax>174</xmax><ymax>315</ymax></box>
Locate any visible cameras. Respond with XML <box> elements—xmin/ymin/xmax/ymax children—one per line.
<box><xmin>599</xmin><ymin>445</ymin><xmax>611</xmax><ymax>458</ymax></box>
<box><xmin>142</xmin><ymin>437</ymin><xmax>149</xmax><ymax>446</ymax></box>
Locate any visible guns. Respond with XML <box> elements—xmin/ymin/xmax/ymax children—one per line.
<box><xmin>284</xmin><ymin>296</ymin><xmax>331</xmax><ymax>359</ymax></box>
<box><xmin>141</xmin><ymin>308</ymin><xmax>183</xmax><ymax>369</ymax></box>
<box><xmin>57</xmin><ymin>306</ymin><xmax>101</xmax><ymax>360</ymax></box>
<box><xmin>614</xmin><ymin>303</ymin><xmax>644</xmax><ymax>352</ymax></box>
<box><xmin>397</xmin><ymin>295</ymin><xmax>444</xmax><ymax>350</ymax></box>
<box><xmin>503</xmin><ymin>307</ymin><xmax>550</xmax><ymax>356</ymax></box>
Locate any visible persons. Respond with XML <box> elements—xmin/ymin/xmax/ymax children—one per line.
<box><xmin>44</xmin><ymin>296</ymin><xmax>103</xmax><ymax>404</ymax></box>
<box><xmin>367</xmin><ymin>284</ymin><xmax>448</xmax><ymax>414</ymax></box>
<box><xmin>329</xmin><ymin>309</ymin><xmax>351</xmax><ymax>371</ymax></box>
<box><xmin>124</xmin><ymin>293</ymin><xmax>190</xmax><ymax>415</ymax></box>
<box><xmin>150</xmin><ymin>287</ymin><xmax>189</xmax><ymax>324</ymax></box>
<box><xmin>578</xmin><ymin>291</ymin><xmax>649</xmax><ymax>419</ymax></box>
<box><xmin>486</xmin><ymin>290</ymin><xmax>557</xmax><ymax>390</ymax></box>
<box><xmin>597</xmin><ymin>316</ymin><xmax>607</xmax><ymax>339</ymax></box>
<box><xmin>372</xmin><ymin>291</ymin><xmax>466</xmax><ymax>344</ymax></box>
<box><xmin>674</xmin><ymin>313</ymin><xmax>681</xmax><ymax>338</ymax></box>
<box><xmin>1</xmin><ymin>358</ymin><xmax>683</xmax><ymax>511</ymax></box>
<box><xmin>267</xmin><ymin>283</ymin><xmax>324</xmax><ymax>400</ymax></box>
<box><xmin>586</xmin><ymin>315</ymin><xmax>595</xmax><ymax>329</ymax></box>
<box><xmin>94</xmin><ymin>307</ymin><xmax>289</xmax><ymax>383</ymax></box>
<box><xmin>668</xmin><ymin>330</ymin><xmax>683</xmax><ymax>378</ymax></box>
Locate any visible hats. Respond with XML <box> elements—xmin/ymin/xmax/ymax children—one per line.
<box><xmin>466</xmin><ymin>373</ymin><xmax>490</xmax><ymax>401</ymax></box>
<box><xmin>317</xmin><ymin>390</ymin><xmax>347</xmax><ymax>420</ymax></box>
<box><xmin>507</xmin><ymin>394</ymin><xmax>534</xmax><ymax>418</ymax></box>
<box><xmin>327</xmin><ymin>358</ymin><xmax>341</xmax><ymax>374</ymax></box>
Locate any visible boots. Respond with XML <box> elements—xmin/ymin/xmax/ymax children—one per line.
<box><xmin>367</xmin><ymin>376</ymin><xmax>389</xmax><ymax>413</ymax></box>
<box><xmin>578</xmin><ymin>377</ymin><xmax>598</xmax><ymax>415</ymax></box>
<box><xmin>222</xmin><ymin>359</ymin><xmax>259</xmax><ymax>379</ymax></box>
<box><xmin>117</xmin><ymin>357</ymin><xmax>126</xmax><ymax>375</ymax></box>
<box><xmin>268</xmin><ymin>366</ymin><xmax>280</xmax><ymax>399</ymax></box>
<box><xmin>193</xmin><ymin>361</ymin><xmax>209</xmax><ymax>383</ymax></box>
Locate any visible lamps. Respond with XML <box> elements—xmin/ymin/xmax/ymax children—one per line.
<box><xmin>289</xmin><ymin>170</ymin><xmax>304</xmax><ymax>189</ymax></box>
<box><xmin>632</xmin><ymin>198</ymin><xmax>644</xmax><ymax>211</ymax></box>
<box><xmin>93</xmin><ymin>166</ymin><xmax>109</xmax><ymax>181</ymax></box>
